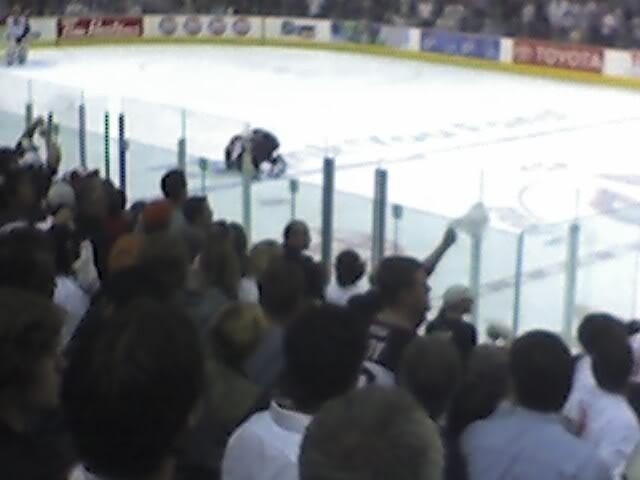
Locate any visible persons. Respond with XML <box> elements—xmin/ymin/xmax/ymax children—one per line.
<box><xmin>5</xmin><ymin>6</ymin><xmax>33</xmax><ymax>65</ymax></box>
<box><xmin>1</xmin><ymin>1</ymin><xmax>640</xmax><ymax>46</ymax></box>
<box><xmin>3</xmin><ymin>116</ymin><xmax>639</xmax><ymax>478</ymax></box>
